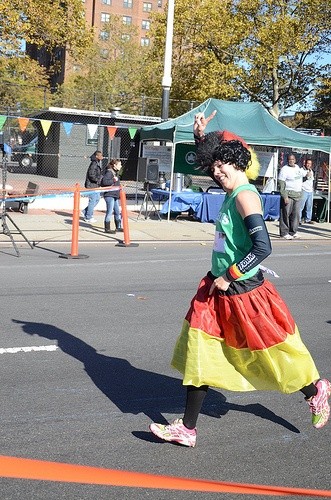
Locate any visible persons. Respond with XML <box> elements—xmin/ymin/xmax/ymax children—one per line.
<box><xmin>81</xmin><ymin>151</ymin><xmax>104</xmax><ymax>223</ymax></box>
<box><xmin>100</xmin><ymin>159</ymin><xmax>124</xmax><ymax>233</ymax></box>
<box><xmin>278</xmin><ymin>153</ymin><xmax>303</xmax><ymax>240</ymax></box>
<box><xmin>149</xmin><ymin>111</ymin><xmax>331</xmax><ymax>448</ymax></box>
<box><xmin>299</xmin><ymin>159</ymin><xmax>315</xmax><ymax>225</ymax></box>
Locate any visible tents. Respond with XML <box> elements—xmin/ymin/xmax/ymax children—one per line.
<box><xmin>135</xmin><ymin>98</ymin><xmax>331</xmax><ymax>223</ymax></box>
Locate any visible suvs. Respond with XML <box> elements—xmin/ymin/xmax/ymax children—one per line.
<box><xmin>11</xmin><ymin>136</ymin><xmax>38</xmax><ymax>170</ymax></box>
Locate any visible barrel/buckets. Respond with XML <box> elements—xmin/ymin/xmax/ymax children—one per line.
<box><xmin>173</xmin><ymin>172</ymin><xmax>184</xmax><ymax>192</ymax></box>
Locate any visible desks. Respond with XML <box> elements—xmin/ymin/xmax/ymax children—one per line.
<box><xmin>151</xmin><ymin>189</ymin><xmax>281</xmax><ymax>223</ymax></box>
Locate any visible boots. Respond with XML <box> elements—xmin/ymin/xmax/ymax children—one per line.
<box><xmin>115</xmin><ymin>219</ymin><xmax>123</xmax><ymax>232</ymax></box>
<box><xmin>105</xmin><ymin>221</ymin><xmax>115</xmax><ymax>234</ymax></box>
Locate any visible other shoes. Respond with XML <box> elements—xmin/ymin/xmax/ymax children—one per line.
<box><xmin>82</xmin><ymin>210</ymin><xmax>89</xmax><ymax>222</ymax></box>
<box><xmin>290</xmin><ymin>232</ymin><xmax>300</xmax><ymax>239</ymax></box>
<box><xmin>280</xmin><ymin>233</ymin><xmax>293</xmax><ymax>240</ymax></box>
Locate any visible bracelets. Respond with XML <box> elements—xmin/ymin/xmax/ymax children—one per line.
<box><xmin>193</xmin><ymin>134</ymin><xmax>204</xmax><ymax>141</ymax></box>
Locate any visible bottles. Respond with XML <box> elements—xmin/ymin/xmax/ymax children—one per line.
<box><xmin>158</xmin><ymin>171</ymin><xmax>166</xmax><ymax>188</ymax></box>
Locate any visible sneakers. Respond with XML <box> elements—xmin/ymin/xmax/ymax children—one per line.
<box><xmin>149</xmin><ymin>418</ymin><xmax>196</xmax><ymax>447</ymax></box>
<box><xmin>304</xmin><ymin>379</ymin><xmax>331</xmax><ymax>429</ymax></box>
